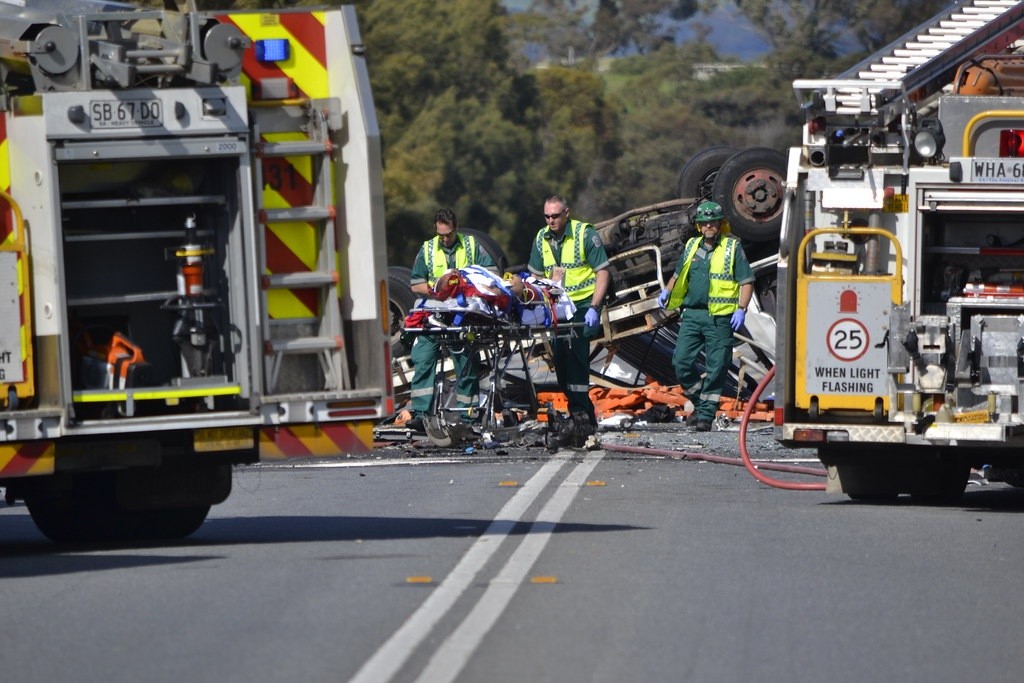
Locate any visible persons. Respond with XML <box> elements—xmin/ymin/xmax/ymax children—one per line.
<box><xmin>404</xmin><ymin>207</ymin><xmax>501</xmax><ymax>429</ymax></box>
<box><xmin>658</xmin><ymin>201</ymin><xmax>756</xmax><ymax>431</ymax></box>
<box><xmin>429</xmin><ymin>267</ymin><xmax>565</xmax><ymax>311</ymax></box>
<box><xmin>527</xmin><ymin>193</ymin><xmax>612</xmax><ymax>428</ymax></box>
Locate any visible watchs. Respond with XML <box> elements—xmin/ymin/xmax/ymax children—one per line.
<box><xmin>590</xmin><ymin>304</ymin><xmax>599</xmax><ymax>313</ymax></box>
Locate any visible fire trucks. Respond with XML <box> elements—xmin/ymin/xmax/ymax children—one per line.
<box><xmin>0</xmin><ymin>0</ymin><xmax>397</xmax><ymax>539</ymax></box>
<box><xmin>775</xmin><ymin>0</ymin><xmax>1024</xmax><ymax>510</ymax></box>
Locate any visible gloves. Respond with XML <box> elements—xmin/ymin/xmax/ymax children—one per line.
<box><xmin>658</xmin><ymin>289</ymin><xmax>670</xmax><ymax>309</ymax></box>
<box><xmin>730</xmin><ymin>309</ymin><xmax>745</xmax><ymax>331</ymax></box>
<box><xmin>584</xmin><ymin>308</ymin><xmax>599</xmax><ymax>327</ymax></box>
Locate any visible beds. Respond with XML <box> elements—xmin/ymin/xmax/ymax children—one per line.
<box><xmin>405</xmin><ymin>322</ymin><xmax>578</xmax><ymax>431</ymax></box>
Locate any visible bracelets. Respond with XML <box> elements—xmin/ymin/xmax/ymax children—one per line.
<box><xmin>738</xmin><ymin>306</ymin><xmax>747</xmax><ymax>312</ymax></box>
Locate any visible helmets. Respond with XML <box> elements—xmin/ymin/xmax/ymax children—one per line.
<box><xmin>695</xmin><ymin>201</ymin><xmax>723</xmax><ymax>221</ymax></box>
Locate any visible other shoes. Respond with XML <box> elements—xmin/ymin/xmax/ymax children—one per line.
<box><xmin>570</xmin><ymin>420</ymin><xmax>598</xmax><ymax>438</ymax></box>
<box><xmin>686</xmin><ymin>409</ymin><xmax>698</xmax><ymax>426</ymax></box>
<box><xmin>696</xmin><ymin>419</ymin><xmax>711</xmax><ymax>431</ymax></box>
<box><xmin>405</xmin><ymin>418</ymin><xmax>425</xmax><ymax>432</ymax></box>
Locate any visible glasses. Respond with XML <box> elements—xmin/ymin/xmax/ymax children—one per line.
<box><xmin>435</xmin><ymin>225</ymin><xmax>456</xmax><ymax>238</ymax></box>
<box><xmin>543</xmin><ymin>209</ymin><xmax>567</xmax><ymax>219</ymax></box>
<box><xmin>698</xmin><ymin>220</ymin><xmax>721</xmax><ymax>225</ymax></box>
